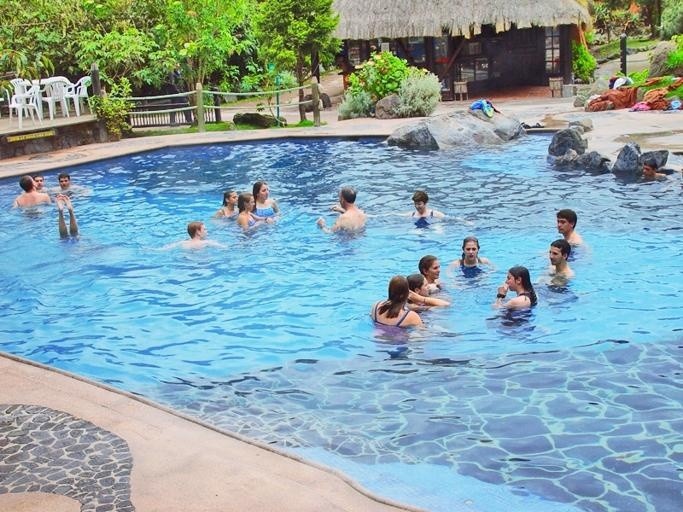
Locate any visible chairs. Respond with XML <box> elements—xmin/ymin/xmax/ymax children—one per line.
<box><xmin>0</xmin><ymin>75</ymin><xmax>91</xmax><ymax>128</ymax></box>
<box><xmin>453</xmin><ymin>80</ymin><xmax>469</xmax><ymax>101</ymax></box>
<box><xmin>549</xmin><ymin>77</ymin><xmax>564</xmax><ymax>97</ymax></box>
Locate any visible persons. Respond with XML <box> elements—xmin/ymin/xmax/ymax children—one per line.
<box><xmin>169</xmin><ymin>63</ymin><xmax>192</xmax><ymax>123</ymax></box>
<box><xmin>54</xmin><ymin>194</ymin><xmax>79</xmax><ymax>237</ymax></box>
<box><xmin>12</xmin><ymin>173</ymin><xmax>83</xmax><ymax>208</ymax></box>
<box><xmin>166</xmin><ymin>182</ymin><xmax>279</xmax><ymax>254</ymax></box>
<box><xmin>369</xmin><ymin>236</ymin><xmax>575</xmax><ymax>332</ymax></box>
<box><xmin>379</xmin><ymin>191</ymin><xmax>473</xmax><ymax>234</ymax></box>
<box><xmin>641</xmin><ymin>159</ymin><xmax>668</xmax><ymax>181</ymax></box>
<box><xmin>555</xmin><ymin>209</ymin><xmax>584</xmax><ymax>246</ymax></box>
<box><xmin>317</xmin><ymin>187</ymin><xmax>366</xmax><ymax>236</ymax></box>
